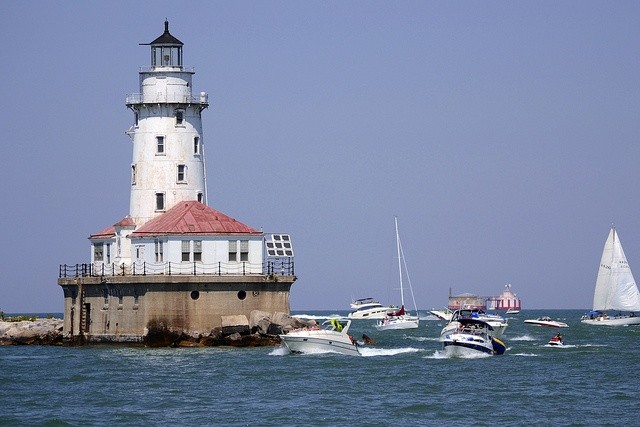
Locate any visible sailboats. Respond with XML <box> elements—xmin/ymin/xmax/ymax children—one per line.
<box><xmin>580</xmin><ymin>224</ymin><xmax>640</xmax><ymax>325</ymax></box>
<box><xmin>375</xmin><ymin>215</ymin><xmax>420</xmax><ymax>331</ymax></box>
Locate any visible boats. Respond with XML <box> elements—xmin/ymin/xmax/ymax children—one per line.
<box><xmin>279</xmin><ymin>318</ymin><xmax>363</xmax><ymax>356</ymax></box>
<box><xmin>505</xmin><ymin>308</ymin><xmax>520</xmax><ymax>314</ymax></box>
<box><xmin>523</xmin><ymin>315</ymin><xmax>570</xmax><ymax>328</ymax></box>
<box><xmin>429</xmin><ymin>307</ymin><xmax>453</xmax><ymax>323</ymax></box>
<box><xmin>448</xmin><ymin>306</ymin><xmax>509</xmax><ymax>339</ymax></box>
<box><xmin>548</xmin><ymin>337</ymin><xmax>564</xmax><ymax>345</ymax></box>
<box><xmin>348</xmin><ymin>296</ymin><xmax>402</xmax><ymax>319</ymax></box>
<box><xmin>440</xmin><ymin>319</ymin><xmax>509</xmax><ymax>358</ymax></box>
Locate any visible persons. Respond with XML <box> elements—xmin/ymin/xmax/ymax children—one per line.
<box><xmin>588</xmin><ymin>314</ymin><xmax>609</xmax><ymax>322</ymax></box>
<box><xmin>555</xmin><ymin>333</ymin><xmax>563</xmax><ymax>341</ymax></box>
